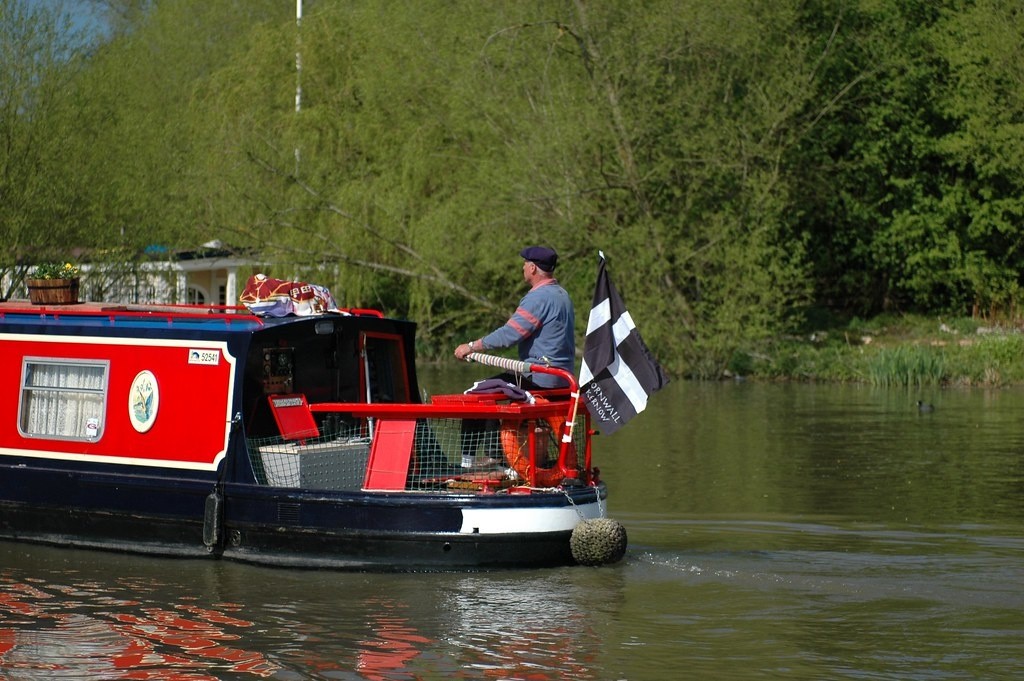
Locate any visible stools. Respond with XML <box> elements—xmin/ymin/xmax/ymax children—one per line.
<box><xmin>431</xmin><ymin>388</ymin><xmax>570</xmax><ymax>405</ymax></box>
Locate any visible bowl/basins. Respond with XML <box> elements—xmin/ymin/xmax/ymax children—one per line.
<box><xmin>26</xmin><ymin>278</ymin><xmax>79</xmax><ymax>305</ymax></box>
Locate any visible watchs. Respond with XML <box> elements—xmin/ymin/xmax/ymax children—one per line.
<box><xmin>468</xmin><ymin>341</ymin><xmax>475</xmax><ymax>354</ymax></box>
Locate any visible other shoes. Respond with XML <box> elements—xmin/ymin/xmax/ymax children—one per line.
<box><xmin>477</xmin><ymin>457</ymin><xmax>503</xmax><ymax>467</ymax></box>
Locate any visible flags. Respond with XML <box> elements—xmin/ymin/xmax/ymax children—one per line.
<box><xmin>578</xmin><ymin>257</ymin><xmax>670</xmax><ymax>436</ymax></box>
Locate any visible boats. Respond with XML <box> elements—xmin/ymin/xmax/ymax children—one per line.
<box><xmin>0</xmin><ymin>246</ymin><xmax>622</xmax><ymax>569</ymax></box>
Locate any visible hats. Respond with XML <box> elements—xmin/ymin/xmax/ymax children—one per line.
<box><xmin>520</xmin><ymin>246</ymin><xmax>557</xmax><ymax>271</ymax></box>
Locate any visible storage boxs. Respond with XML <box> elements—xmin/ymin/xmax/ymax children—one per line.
<box><xmin>258</xmin><ymin>441</ymin><xmax>369</xmax><ymax>490</ymax></box>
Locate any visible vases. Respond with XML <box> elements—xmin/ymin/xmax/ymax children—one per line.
<box><xmin>25</xmin><ymin>277</ymin><xmax>81</xmax><ymax>305</ymax></box>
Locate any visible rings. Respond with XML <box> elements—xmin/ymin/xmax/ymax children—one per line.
<box><xmin>455</xmin><ymin>353</ymin><xmax>457</xmax><ymax>355</ymax></box>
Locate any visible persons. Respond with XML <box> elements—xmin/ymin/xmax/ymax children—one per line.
<box><xmin>444</xmin><ymin>242</ymin><xmax>575</xmax><ymax>480</ymax></box>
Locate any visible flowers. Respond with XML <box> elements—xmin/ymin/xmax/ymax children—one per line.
<box><xmin>30</xmin><ymin>261</ymin><xmax>78</xmax><ymax>280</ymax></box>
